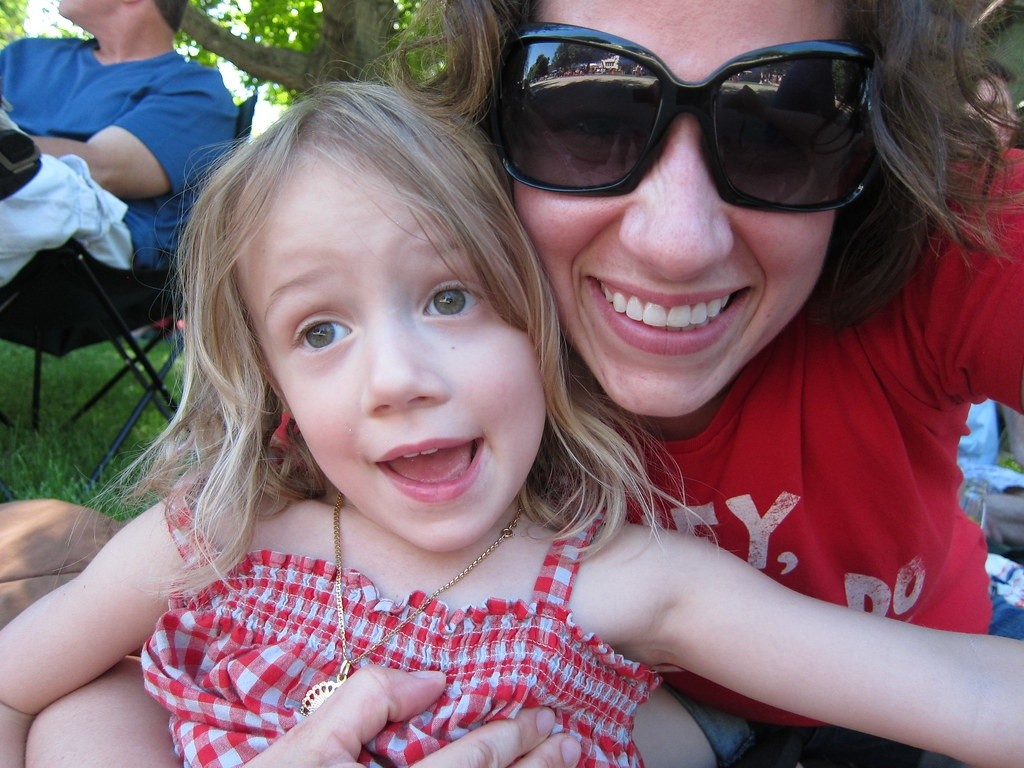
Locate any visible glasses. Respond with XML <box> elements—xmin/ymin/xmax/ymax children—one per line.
<box><xmin>486</xmin><ymin>21</ymin><xmax>891</xmax><ymax>213</ymax></box>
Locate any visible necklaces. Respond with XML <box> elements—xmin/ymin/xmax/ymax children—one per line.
<box><xmin>299</xmin><ymin>492</ymin><xmax>527</xmax><ymax>718</ymax></box>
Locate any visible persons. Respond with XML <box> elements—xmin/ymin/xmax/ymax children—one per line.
<box><xmin>0</xmin><ymin>0</ymin><xmax>242</xmax><ymax>359</ymax></box>
<box><xmin>543</xmin><ymin>123</ymin><xmax>639</xmax><ymax>188</ymax></box>
<box><xmin>1</xmin><ymin>58</ymin><xmax>1022</xmax><ymax>768</ymax></box>
<box><xmin>764</xmin><ymin>55</ymin><xmax>839</xmax><ymax>167</ymax></box>
<box><xmin>21</xmin><ymin>0</ymin><xmax>1023</xmax><ymax>768</ymax></box>
<box><xmin>957</xmin><ymin>67</ymin><xmax>1022</xmax><ymax>558</ymax></box>
<box><xmin>721</xmin><ymin>83</ymin><xmax>876</xmax><ymax>205</ymax></box>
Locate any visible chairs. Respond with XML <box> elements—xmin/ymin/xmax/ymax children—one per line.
<box><xmin>1</xmin><ymin>94</ymin><xmax>254</xmax><ymax>490</ymax></box>
